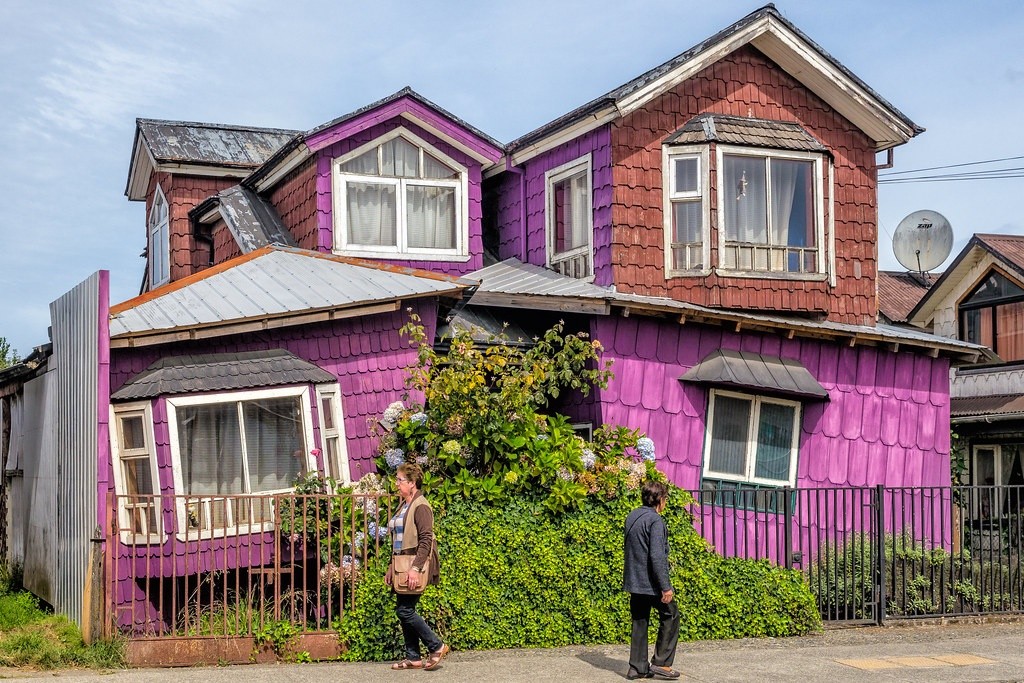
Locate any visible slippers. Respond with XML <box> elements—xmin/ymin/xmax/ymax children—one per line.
<box><xmin>426</xmin><ymin>643</ymin><xmax>449</xmax><ymax>670</ymax></box>
<box><xmin>393</xmin><ymin>659</ymin><xmax>423</xmax><ymax>669</ymax></box>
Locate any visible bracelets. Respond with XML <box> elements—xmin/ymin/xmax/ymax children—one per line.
<box><xmin>412</xmin><ymin>566</ymin><xmax>420</xmax><ymax>572</ymax></box>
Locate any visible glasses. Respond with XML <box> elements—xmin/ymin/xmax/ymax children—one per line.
<box><xmin>396</xmin><ymin>476</ymin><xmax>412</xmax><ymax>483</ymax></box>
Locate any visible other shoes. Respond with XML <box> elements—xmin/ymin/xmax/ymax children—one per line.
<box><xmin>649</xmin><ymin>664</ymin><xmax>680</xmax><ymax>677</ymax></box>
<box><xmin>627</xmin><ymin>668</ymin><xmax>654</xmax><ymax>680</ymax></box>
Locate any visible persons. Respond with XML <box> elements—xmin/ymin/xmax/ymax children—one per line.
<box><xmin>384</xmin><ymin>464</ymin><xmax>448</xmax><ymax>671</ymax></box>
<box><xmin>624</xmin><ymin>479</ymin><xmax>682</xmax><ymax>680</ymax></box>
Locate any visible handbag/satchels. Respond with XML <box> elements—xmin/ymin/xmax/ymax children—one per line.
<box><xmin>392</xmin><ymin>555</ymin><xmax>430</xmax><ymax>594</ymax></box>
